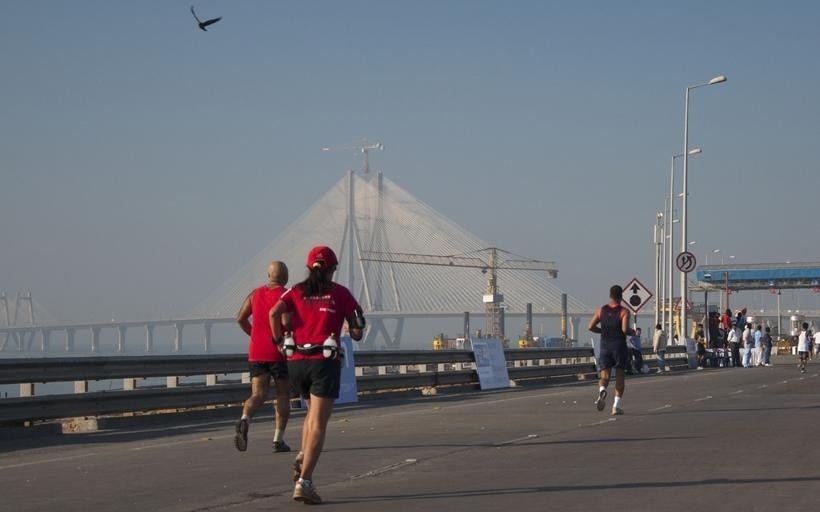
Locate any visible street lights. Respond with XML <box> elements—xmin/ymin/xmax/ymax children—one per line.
<box><xmin>681</xmin><ymin>75</ymin><xmax>728</xmax><ymax>341</ymax></box>
<box><xmin>654</xmin><ymin>149</ymin><xmax>738</xmax><ymax>333</ymax></box>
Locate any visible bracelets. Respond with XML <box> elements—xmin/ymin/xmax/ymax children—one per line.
<box><xmin>272</xmin><ymin>337</ymin><xmax>283</xmax><ymax>345</ymax></box>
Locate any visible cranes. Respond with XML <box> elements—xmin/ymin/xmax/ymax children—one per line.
<box><xmin>322</xmin><ymin>132</ymin><xmax>386</xmax><ymax>177</ymax></box>
<box><xmin>360</xmin><ymin>246</ymin><xmax>559</xmax><ymax>338</ymax></box>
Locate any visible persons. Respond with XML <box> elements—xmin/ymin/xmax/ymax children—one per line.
<box><xmin>269</xmin><ymin>246</ymin><xmax>365</xmax><ymax>504</ymax></box>
<box><xmin>589</xmin><ymin>285</ymin><xmax>636</xmax><ymax>414</ymax></box>
<box><xmin>672</xmin><ymin>334</ymin><xmax>678</xmax><ymax>347</ymax></box>
<box><xmin>234</xmin><ymin>261</ymin><xmax>291</xmax><ymax>452</ymax></box>
<box><xmin>795</xmin><ymin>321</ymin><xmax>820</xmax><ymax>374</ymax></box>
<box><xmin>694</xmin><ymin>306</ymin><xmax>772</xmax><ymax>371</ymax></box>
<box><xmin>629</xmin><ymin>328</ymin><xmax>643</xmax><ymax>375</ymax></box>
<box><xmin>653</xmin><ymin>324</ymin><xmax>667</xmax><ymax>374</ymax></box>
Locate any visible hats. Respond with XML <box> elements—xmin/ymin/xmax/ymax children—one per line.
<box><xmin>306</xmin><ymin>245</ymin><xmax>339</xmax><ymax>271</ymax></box>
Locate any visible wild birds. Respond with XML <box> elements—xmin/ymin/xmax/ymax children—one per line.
<box><xmin>190</xmin><ymin>4</ymin><xmax>223</xmax><ymax>32</ymax></box>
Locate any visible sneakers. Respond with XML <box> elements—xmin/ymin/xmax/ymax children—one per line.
<box><xmin>595</xmin><ymin>386</ymin><xmax>607</xmax><ymax>411</ymax></box>
<box><xmin>272</xmin><ymin>440</ymin><xmax>291</xmax><ymax>453</ymax></box>
<box><xmin>292</xmin><ymin>478</ymin><xmax>321</xmax><ymax>504</ymax></box>
<box><xmin>612</xmin><ymin>408</ymin><xmax>624</xmax><ymax>415</ymax></box>
<box><xmin>234</xmin><ymin>419</ymin><xmax>249</xmax><ymax>451</ymax></box>
<box><xmin>293</xmin><ymin>451</ymin><xmax>304</xmax><ymax>481</ymax></box>
